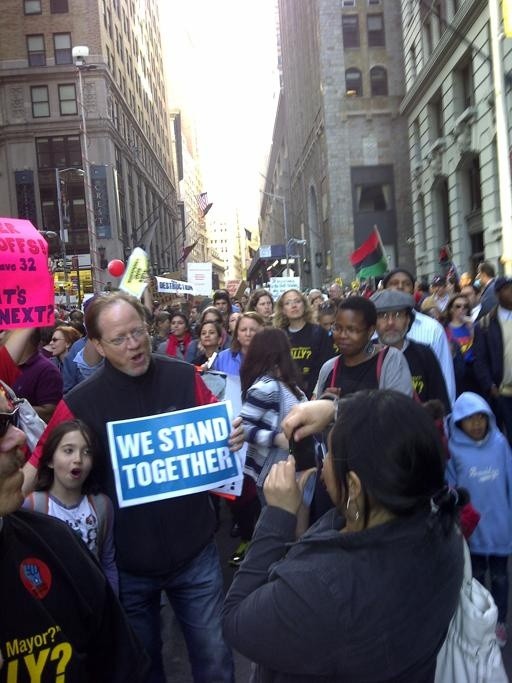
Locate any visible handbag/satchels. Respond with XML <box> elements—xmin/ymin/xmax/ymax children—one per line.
<box><xmin>435</xmin><ymin>575</ymin><xmax>509</xmax><ymax>683</ymax></box>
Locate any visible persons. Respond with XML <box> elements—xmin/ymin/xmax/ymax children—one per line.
<box><xmin>0</xmin><ymin>263</ymin><xmax>512</xmax><ymax>683</ymax></box>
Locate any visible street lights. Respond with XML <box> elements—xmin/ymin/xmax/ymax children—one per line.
<box><xmin>259</xmin><ymin>184</ymin><xmax>289</xmax><ymax>276</ymax></box>
<box><xmin>39</xmin><ymin>166</ymin><xmax>85</xmax><ymax>312</ymax></box>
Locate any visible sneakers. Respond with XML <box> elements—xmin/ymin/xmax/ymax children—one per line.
<box><xmin>229</xmin><ymin>541</ymin><xmax>249</xmax><ymax>565</ymax></box>
<box><xmin>231</xmin><ymin>521</ymin><xmax>239</xmax><ymax>538</ymax></box>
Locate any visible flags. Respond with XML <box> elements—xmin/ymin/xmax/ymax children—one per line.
<box><xmin>348</xmin><ymin>231</ymin><xmax>387</xmax><ymax>278</ymax></box>
<box><xmin>195</xmin><ymin>192</ymin><xmax>208</xmax><ymax>211</ymax></box>
<box><xmin>203</xmin><ymin>204</ymin><xmax>212</xmax><ymax>217</ymax></box>
<box><xmin>177</xmin><ymin>244</ymin><xmax>196</xmax><ymax>263</ymax></box>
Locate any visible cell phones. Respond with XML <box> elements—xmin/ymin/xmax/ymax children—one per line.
<box><xmin>207</xmin><ymin>351</ymin><xmax>217</xmax><ymax>369</ymax></box>
<box><xmin>288</xmin><ymin>425</ymin><xmax>316</xmax><ymax>473</ymax></box>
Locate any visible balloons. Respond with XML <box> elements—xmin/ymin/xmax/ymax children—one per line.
<box><xmin>108</xmin><ymin>259</ymin><xmax>125</xmax><ymax>277</ymax></box>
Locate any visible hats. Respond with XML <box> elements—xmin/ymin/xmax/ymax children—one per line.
<box><xmin>432</xmin><ymin>275</ymin><xmax>446</xmax><ymax>287</ymax></box>
<box><xmin>368</xmin><ymin>287</ymin><xmax>414</xmax><ymax>313</ymax></box>
<box><xmin>383</xmin><ymin>269</ymin><xmax>415</xmax><ymax>288</ymax></box>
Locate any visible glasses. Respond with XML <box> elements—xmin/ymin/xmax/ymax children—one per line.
<box><xmin>112</xmin><ymin>325</ymin><xmax>146</xmax><ymax>345</ymax></box>
<box><xmin>456</xmin><ymin>304</ymin><xmax>469</xmax><ymax>309</ymax></box>
<box><xmin>379</xmin><ymin>309</ymin><xmax>405</xmax><ymax>319</ymax></box>
<box><xmin>0</xmin><ymin>407</ymin><xmax>20</xmax><ymax>439</ymax></box>
<box><xmin>330</xmin><ymin>324</ymin><xmax>369</xmax><ymax>334</ymax></box>
<box><xmin>50</xmin><ymin>338</ymin><xmax>65</xmax><ymax>341</ymax></box>
